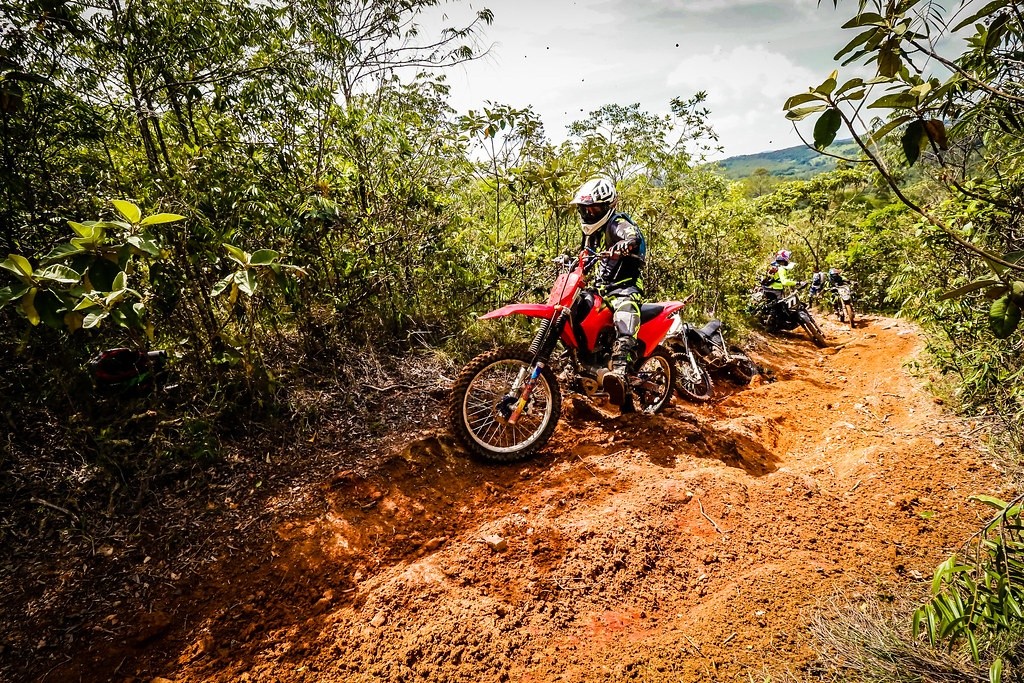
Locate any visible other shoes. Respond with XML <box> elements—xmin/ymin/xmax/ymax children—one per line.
<box><xmin>602</xmin><ymin>372</ymin><xmax>625</xmax><ymax>406</ymax></box>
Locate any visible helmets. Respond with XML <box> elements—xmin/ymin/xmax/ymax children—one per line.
<box><xmin>777</xmin><ymin>249</ymin><xmax>789</xmax><ymax>266</ymax></box>
<box><xmin>829</xmin><ymin>269</ymin><xmax>840</xmax><ymax>278</ymax></box>
<box><xmin>570</xmin><ymin>178</ymin><xmax>618</xmax><ymax>236</ymax></box>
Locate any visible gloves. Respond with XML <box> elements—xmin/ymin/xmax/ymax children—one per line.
<box><xmin>610</xmin><ymin>240</ymin><xmax>637</xmax><ymax>257</ymax></box>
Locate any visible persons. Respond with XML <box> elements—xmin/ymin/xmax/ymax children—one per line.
<box><xmin>555</xmin><ymin>177</ymin><xmax>648</xmax><ymax>406</ymax></box>
<box><xmin>763</xmin><ymin>249</ymin><xmax>807</xmax><ymax>327</ymax></box>
<box><xmin>827</xmin><ymin>271</ymin><xmax>858</xmax><ymax>314</ymax></box>
<box><xmin>807</xmin><ymin>272</ymin><xmax>826</xmax><ymax>308</ymax></box>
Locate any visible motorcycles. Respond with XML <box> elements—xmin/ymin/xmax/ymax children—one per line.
<box><xmin>663</xmin><ymin>316</ymin><xmax>756</xmax><ymax>404</ymax></box>
<box><xmin>448</xmin><ymin>245</ymin><xmax>687</xmax><ymax>466</ymax></box>
<box><xmin>760</xmin><ymin>279</ymin><xmax>858</xmax><ymax>349</ymax></box>
